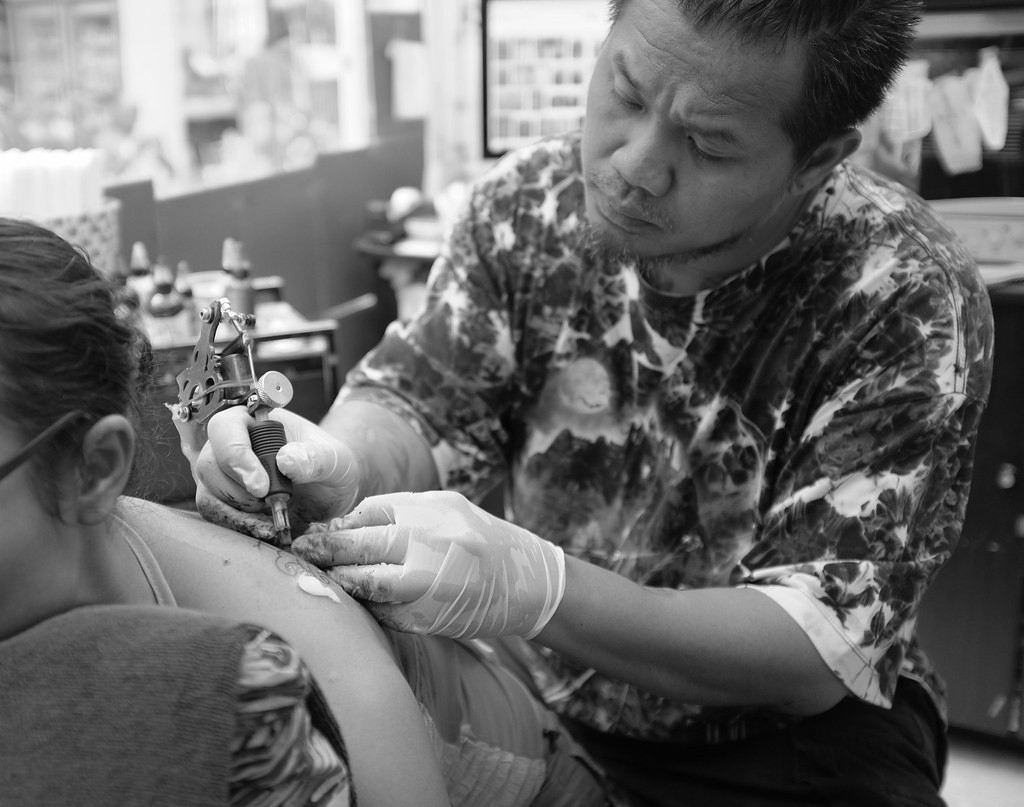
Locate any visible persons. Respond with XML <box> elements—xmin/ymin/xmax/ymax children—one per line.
<box><xmin>194</xmin><ymin>1</ymin><xmax>993</xmax><ymax>807</ymax></box>
<box><xmin>0</xmin><ymin>218</ymin><xmax>624</xmax><ymax>807</ymax></box>
